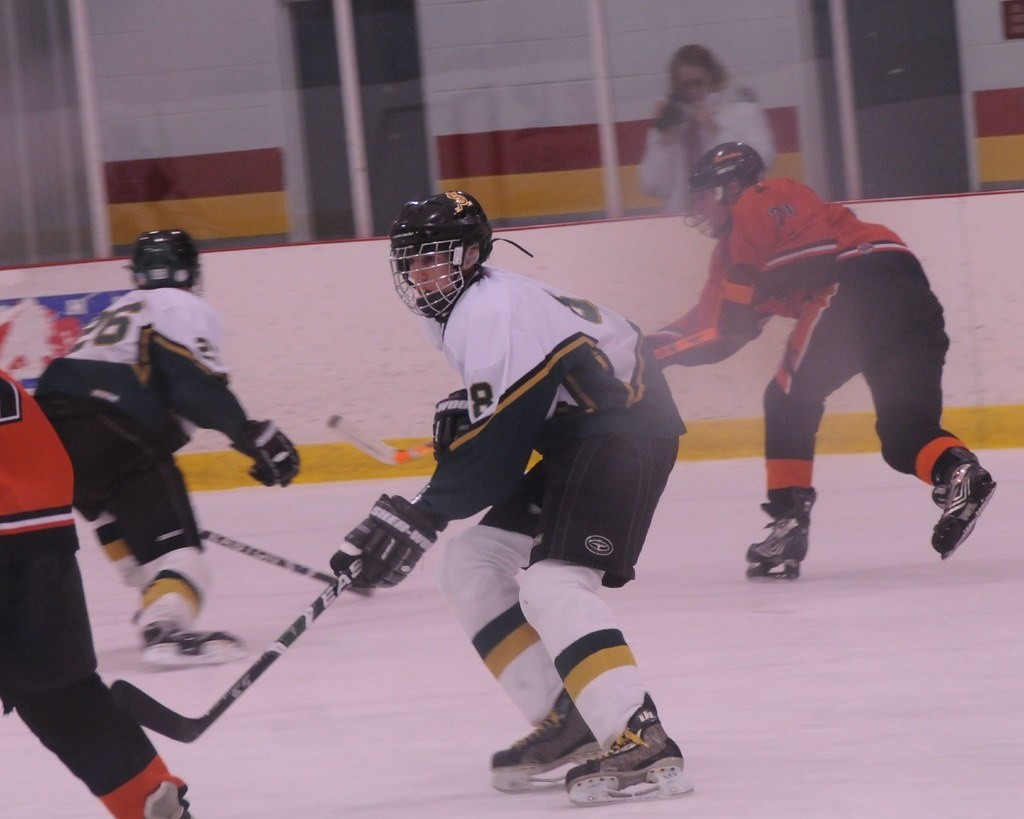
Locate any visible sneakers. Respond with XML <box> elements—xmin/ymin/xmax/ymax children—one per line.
<box><xmin>746</xmin><ymin>490</ymin><xmax>816</xmax><ymax>579</ymax></box>
<box><xmin>492</xmin><ymin>690</ymin><xmax>603</xmax><ymax>791</ymax></box>
<box><xmin>931</xmin><ymin>447</ymin><xmax>997</xmax><ymax>561</ymax></box>
<box><xmin>132</xmin><ymin>609</ymin><xmax>248</xmax><ymax>668</ymax></box>
<box><xmin>566</xmin><ymin>692</ymin><xmax>693</xmax><ymax>805</ymax></box>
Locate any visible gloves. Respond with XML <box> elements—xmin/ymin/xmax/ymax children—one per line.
<box><xmin>640</xmin><ymin>328</ymin><xmax>686</xmax><ymax>369</ymax></box>
<box><xmin>330</xmin><ymin>494</ymin><xmax>437</xmax><ymax>589</ymax></box>
<box><xmin>230</xmin><ymin>419</ymin><xmax>300</xmax><ymax>488</ymax></box>
<box><xmin>432</xmin><ymin>389</ymin><xmax>473</xmax><ymax>459</ymax></box>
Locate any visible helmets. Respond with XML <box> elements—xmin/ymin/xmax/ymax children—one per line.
<box><xmin>683</xmin><ymin>142</ymin><xmax>766</xmax><ymax>238</ymax></box>
<box><xmin>127</xmin><ymin>229</ymin><xmax>198</xmax><ymax>290</ymax></box>
<box><xmin>388</xmin><ymin>191</ymin><xmax>493</xmax><ymax>319</ymax></box>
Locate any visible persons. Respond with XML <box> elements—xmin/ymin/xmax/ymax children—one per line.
<box><xmin>637</xmin><ymin>45</ymin><xmax>774</xmax><ymax>214</ymax></box>
<box><xmin>330</xmin><ymin>193</ymin><xmax>694</xmax><ymax>806</ymax></box>
<box><xmin>0</xmin><ymin>368</ymin><xmax>192</xmax><ymax>819</ymax></box>
<box><xmin>643</xmin><ymin>141</ymin><xmax>998</xmax><ymax>580</ymax></box>
<box><xmin>32</xmin><ymin>227</ymin><xmax>302</xmax><ymax>668</ymax></box>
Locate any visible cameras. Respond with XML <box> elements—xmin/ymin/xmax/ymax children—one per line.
<box><xmin>656</xmin><ymin>95</ymin><xmax>692</xmax><ymax>132</ymax></box>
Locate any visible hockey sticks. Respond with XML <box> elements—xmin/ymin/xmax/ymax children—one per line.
<box><xmin>326</xmin><ymin>414</ymin><xmax>436</xmax><ymax>465</ymax></box>
<box><xmin>197</xmin><ymin>527</ymin><xmax>376</xmax><ymax>590</ymax></box>
<box><xmin>109</xmin><ymin>479</ymin><xmax>431</xmax><ymax>745</ymax></box>
